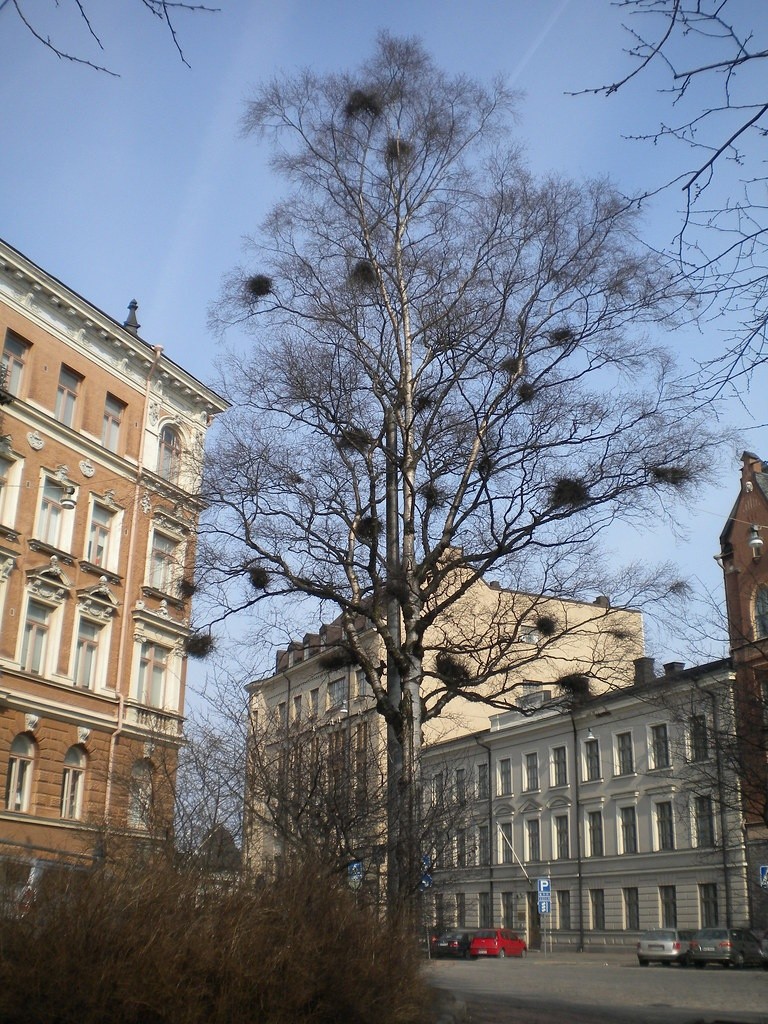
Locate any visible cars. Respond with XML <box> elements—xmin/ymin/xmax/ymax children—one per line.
<box><xmin>688</xmin><ymin>927</ymin><xmax>768</xmax><ymax>970</ymax></box>
<box><xmin>433</xmin><ymin>928</ymin><xmax>482</xmax><ymax>960</ymax></box>
<box><xmin>470</xmin><ymin>928</ymin><xmax>528</xmax><ymax>961</ymax></box>
<box><xmin>635</xmin><ymin>928</ymin><xmax>701</xmax><ymax>968</ymax></box>
